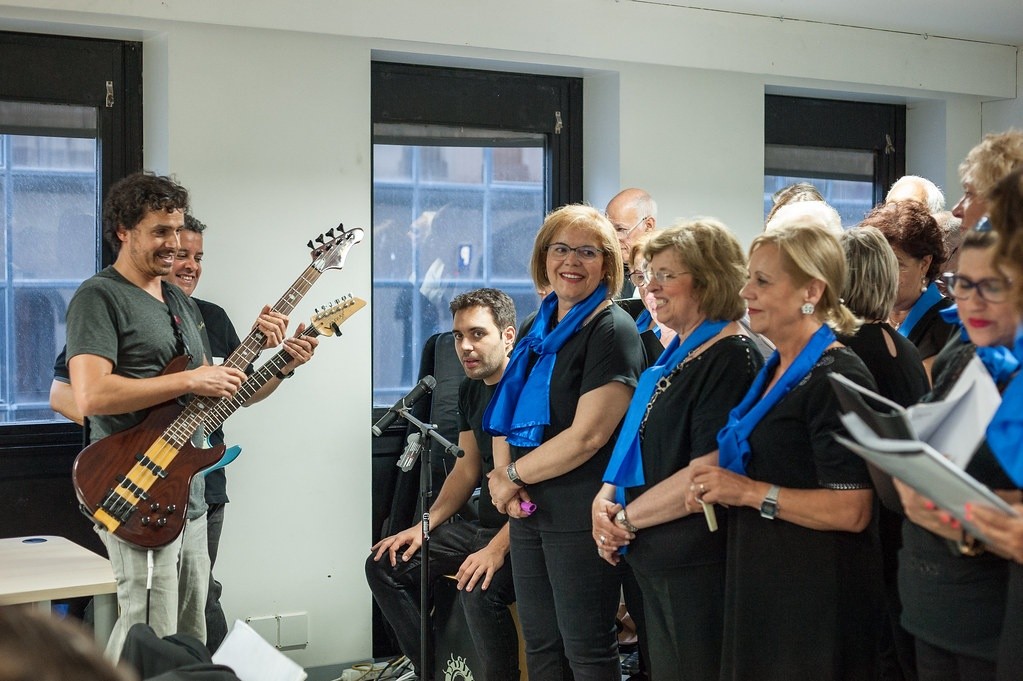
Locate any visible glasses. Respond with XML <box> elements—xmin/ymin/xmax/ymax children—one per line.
<box><xmin>616</xmin><ymin>217</ymin><xmax>648</xmax><ymax>240</ymax></box>
<box><xmin>646</xmin><ymin>269</ymin><xmax>692</xmax><ymax>283</ymax></box>
<box><xmin>944</xmin><ymin>276</ymin><xmax>1008</xmax><ymax>303</ymax></box>
<box><xmin>629</xmin><ymin>271</ymin><xmax>645</xmax><ymax>286</ymax></box>
<box><xmin>545</xmin><ymin>242</ymin><xmax>604</xmax><ymax>261</ymax></box>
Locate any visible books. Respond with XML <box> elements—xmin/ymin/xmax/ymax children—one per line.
<box><xmin>826</xmin><ymin>352</ymin><xmax>1004</xmax><ymax>517</ymax></box>
<box><xmin>833</xmin><ymin>411</ymin><xmax>1018</xmax><ymax>547</ymax></box>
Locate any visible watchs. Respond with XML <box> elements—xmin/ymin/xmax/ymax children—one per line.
<box><xmin>759</xmin><ymin>485</ymin><xmax>781</xmax><ymax>520</ymax></box>
<box><xmin>506</xmin><ymin>458</ymin><xmax>531</xmax><ymax>487</ymax></box>
<box><xmin>616</xmin><ymin>508</ymin><xmax>638</xmax><ymax>532</ymax></box>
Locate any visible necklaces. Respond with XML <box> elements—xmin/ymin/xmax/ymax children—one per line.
<box><xmin>638</xmin><ymin>345</ymin><xmax>703</xmax><ymax>442</ymax></box>
<box><xmin>582</xmin><ymin>317</ymin><xmax>593</xmax><ymax>328</ymax></box>
<box><xmin>890</xmin><ymin>317</ymin><xmax>904</xmax><ymax>331</ymax></box>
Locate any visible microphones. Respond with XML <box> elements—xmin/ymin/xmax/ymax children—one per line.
<box><xmin>372</xmin><ymin>375</ymin><xmax>436</xmax><ymax>436</ymax></box>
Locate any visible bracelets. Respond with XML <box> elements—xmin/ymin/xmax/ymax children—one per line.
<box><xmin>957</xmin><ymin>528</ymin><xmax>983</xmax><ymax>557</ymax></box>
<box><xmin>275</xmin><ymin>369</ymin><xmax>296</xmax><ymax>380</ymax></box>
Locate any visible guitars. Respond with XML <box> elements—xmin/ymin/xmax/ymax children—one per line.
<box><xmin>72</xmin><ymin>223</ymin><xmax>364</xmax><ymax>553</ymax></box>
<box><xmin>201</xmin><ymin>293</ymin><xmax>368</xmax><ymax>478</ymax></box>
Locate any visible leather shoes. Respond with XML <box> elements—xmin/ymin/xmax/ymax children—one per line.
<box><xmin>621</xmin><ymin>652</ymin><xmax>638</xmax><ymax>674</ymax></box>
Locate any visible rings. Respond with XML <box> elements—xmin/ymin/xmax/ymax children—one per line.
<box><xmin>596</xmin><ymin>540</ymin><xmax>603</xmax><ymax>547</ymax></box>
<box><xmin>700</xmin><ymin>484</ymin><xmax>706</xmax><ymax>492</ymax></box>
<box><xmin>600</xmin><ymin>535</ymin><xmax>606</xmax><ymax>542</ymax></box>
<box><xmin>491</xmin><ymin>499</ymin><xmax>497</xmax><ymax>505</ymax></box>
<box><xmin>690</xmin><ymin>485</ymin><xmax>694</xmax><ymax>491</ymax></box>
<box><xmin>598</xmin><ymin>549</ymin><xmax>604</xmax><ymax>556</ymax></box>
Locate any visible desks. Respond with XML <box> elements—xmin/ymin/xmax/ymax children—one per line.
<box><xmin>0</xmin><ymin>533</ymin><xmax>119</xmax><ymax>653</ymax></box>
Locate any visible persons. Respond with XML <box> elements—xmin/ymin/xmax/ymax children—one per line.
<box><xmin>65</xmin><ymin>170</ymin><xmax>289</xmax><ymax>669</ymax></box>
<box><xmin>0</xmin><ymin>605</ymin><xmax>135</xmax><ymax>681</ymax></box>
<box><xmin>488</xmin><ymin>127</ymin><xmax>1023</xmax><ymax>681</ymax></box>
<box><xmin>49</xmin><ymin>214</ymin><xmax>319</xmax><ymax>656</ymax></box>
<box><xmin>365</xmin><ymin>288</ymin><xmax>521</xmax><ymax>681</ymax></box>
